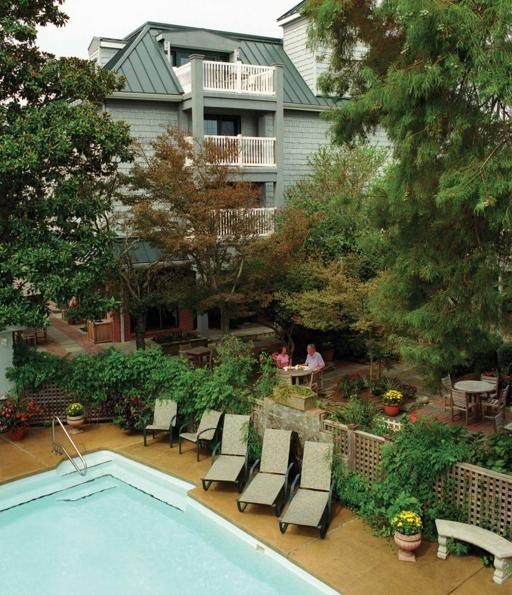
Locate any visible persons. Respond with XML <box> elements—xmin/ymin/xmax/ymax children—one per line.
<box><xmin>300</xmin><ymin>343</ymin><xmax>326</xmax><ymax>392</ymax></box>
<box><xmin>276</xmin><ymin>345</ymin><xmax>290</xmax><ymax>369</ymax></box>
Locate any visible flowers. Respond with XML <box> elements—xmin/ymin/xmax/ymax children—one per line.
<box><xmin>383</xmin><ymin>389</ymin><xmax>403</xmax><ymax>405</ymax></box>
<box><xmin>390</xmin><ymin>510</ymin><xmax>423</xmax><ymax>535</ymax></box>
<box><xmin>0</xmin><ymin>392</ymin><xmax>46</xmax><ymax>433</ymax></box>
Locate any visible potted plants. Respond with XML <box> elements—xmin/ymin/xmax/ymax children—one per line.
<box><xmin>64</xmin><ymin>403</ymin><xmax>86</xmax><ymax>434</ymax></box>
<box><xmin>273</xmin><ymin>384</ymin><xmax>317</xmax><ymax>412</ymax></box>
<box><xmin>112</xmin><ymin>396</ymin><xmax>154</xmax><ymax>433</ymax></box>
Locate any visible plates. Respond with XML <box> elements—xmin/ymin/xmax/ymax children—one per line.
<box><xmin>283</xmin><ymin>364</ymin><xmax>311</xmax><ymax>372</ymax></box>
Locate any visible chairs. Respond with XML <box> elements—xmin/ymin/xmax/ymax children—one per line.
<box><xmin>314</xmin><ymin>368</ymin><xmax>324</xmax><ymax>389</ymax></box>
<box><xmin>235</xmin><ymin>428</ymin><xmax>295</xmax><ymax>517</ymax></box>
<box><xmin>275</xmin><ymin>358</ymin><xmax>292</xmax><ymax>369</ymax></box>
<box><xmin>179</xmin><ymin>408</ymin><xmax>223</xmax><ymax>462</ymax></box>
<box><xmin>143</xmin><ymin>398</ymin><xmax>185</xmax><ymax>448</ymax></box>
<box><xmin>201</xmin><ymin>414</ymin><xmax>251</xmax><ymax>493</ymax></box>
<box><xmin>208</xmin><ymin>343</ymin><xmax>216</xmax><ymax>361</ymax></box>
<box><xmin>440</xmin><ymin>372</ymin><xmax>512</xmax><ymax>434</ymax></box>
<box><xmin>277</xmin><ymin>441</ymin><xmax>337</xmax><ymax>539</ymax></box>
<box><xmin>179</xmin><ymin>342</ymin><xmax>192</xmax><ymax>358</ymax></box>
<box><xmin>12</xmin><ymin>326</ymin><xmax>48</xmax><ymax>348</ymax></box>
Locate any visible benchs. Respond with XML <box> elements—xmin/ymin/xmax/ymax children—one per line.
<box><xmin>434</xmin><ymin>519</ymin><xmax>512</xmax><ymax>584</ymax></box>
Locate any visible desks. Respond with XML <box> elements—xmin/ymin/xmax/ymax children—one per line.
<box><xmin>282</xmin><ymin>365</ymin><xmax>314</xmax><ymax>388</ymax></box>
<box><xmin>184</xmin><ymin>345</ymin><xmax>213</xmax><ymax>369</ymax></box>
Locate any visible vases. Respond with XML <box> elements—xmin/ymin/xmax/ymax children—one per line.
<box><xmin>394</xmin><ymin>530</ymin><xmax>421</xmax><ymax>562</ymax></box>
<box><xmin>6</xmin><ymin>424</ymin><xmax>30</xmax><ymax>441</ymax></box>
<box><xmin>383</xmin><ymin>403</ymin><xmax>401</xmax><ymax>417</ymax></box>
